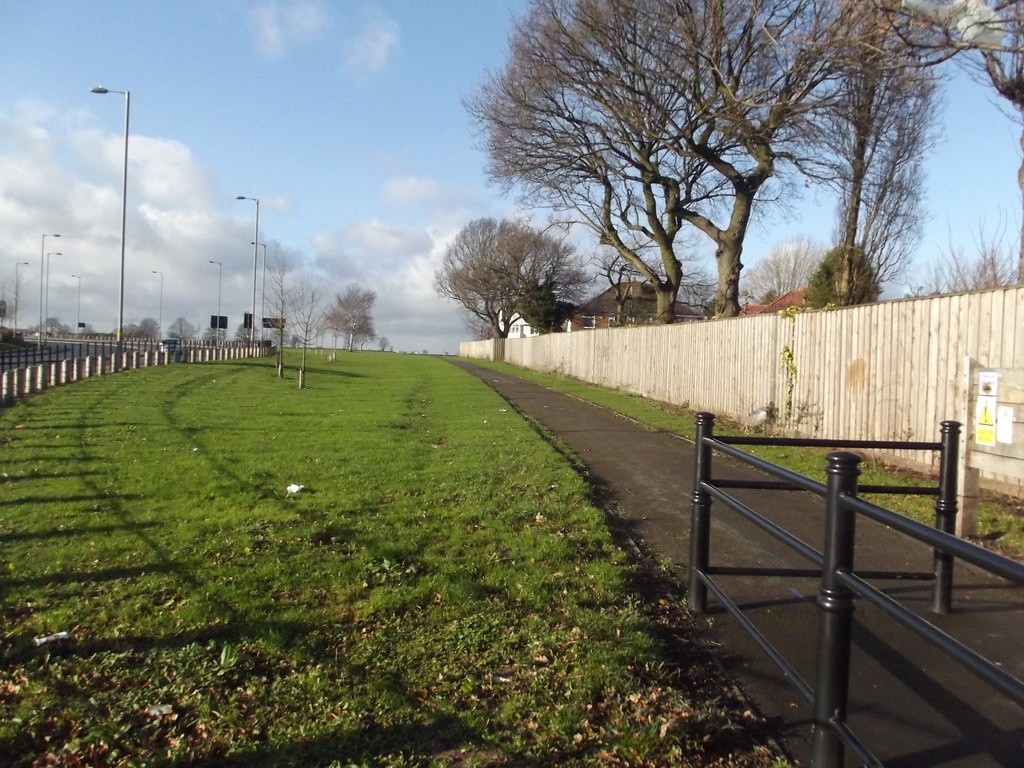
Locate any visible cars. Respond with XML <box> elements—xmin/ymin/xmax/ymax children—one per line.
<box><xmin>15</xmin><ymin>330</ymin><xmax>52</xmax><ymax>339</ymax></box>
<box><xmin>158</xmin><ymin>337</ymin><xmax>183</xmax><ymax>356</ymax></box>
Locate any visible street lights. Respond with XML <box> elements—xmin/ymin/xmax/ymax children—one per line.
<box><xmin>90</xmin><ymin>84</ymin><xmax>131</xmax><ymax>342</ymax></box>
<box><xmin>71</xmin><ymin>275</ymin><xmax>80</xmax><ymax>339</ymax></box>
<box><xmin>15</xmin><ymin>261</ymin><xmax>28</xmax><ymax>338</ymax></box>
<box><xmin>251</xmin><ymin>242</ymin><xmax>266</xmax><ymax>347</ymax></box>
<box><xmin>235</xmin><ymin>195</ymin><xmax>261</xmax><ymax>345</ymax></box>
<box><xmin>44</xmin><ymin>251</ymin><xmax>63</xmax><ymax>347</ymax></box>
<box><xmin>153</xmin><ymin>270</ymin><xmax>162</xmax><ymax>342</ymax></box>
<box><xmin>37</xmin><ymin>234</ymin><xmax>61</xmax><ymax>351</ymax></box>
<box><xmin>209</xmin><ymin>259</ymin><xmax>222</xmax><ymax>348</ymax></box>
